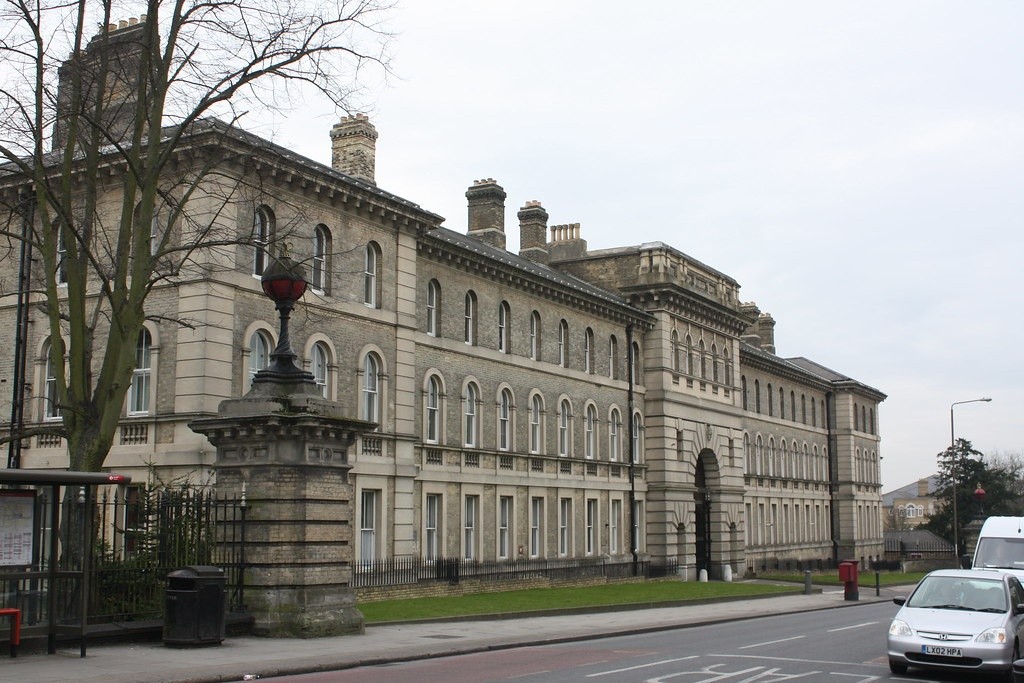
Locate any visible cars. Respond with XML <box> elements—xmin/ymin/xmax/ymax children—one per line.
<box><xmin>887</xmin><ymin>566</ymin><xmax>1024</xmax><ymax>683</ymax></box>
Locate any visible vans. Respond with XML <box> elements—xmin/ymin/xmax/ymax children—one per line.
<box><xmin>970</xmin><ymin>515</ymin><xmax>1024</xmax><ymax>590</ymax></box>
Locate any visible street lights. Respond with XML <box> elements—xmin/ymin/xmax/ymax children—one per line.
<box><xmin>949</xmin><ymin>397</ymin><xmax>993</xmax><ymax>568</ymax></box>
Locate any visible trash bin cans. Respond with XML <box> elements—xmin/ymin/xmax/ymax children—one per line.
<box><xmin>160</xmin><ymin>566</ymin><xmax>229</xmax><ymax>648</ymax></box>
